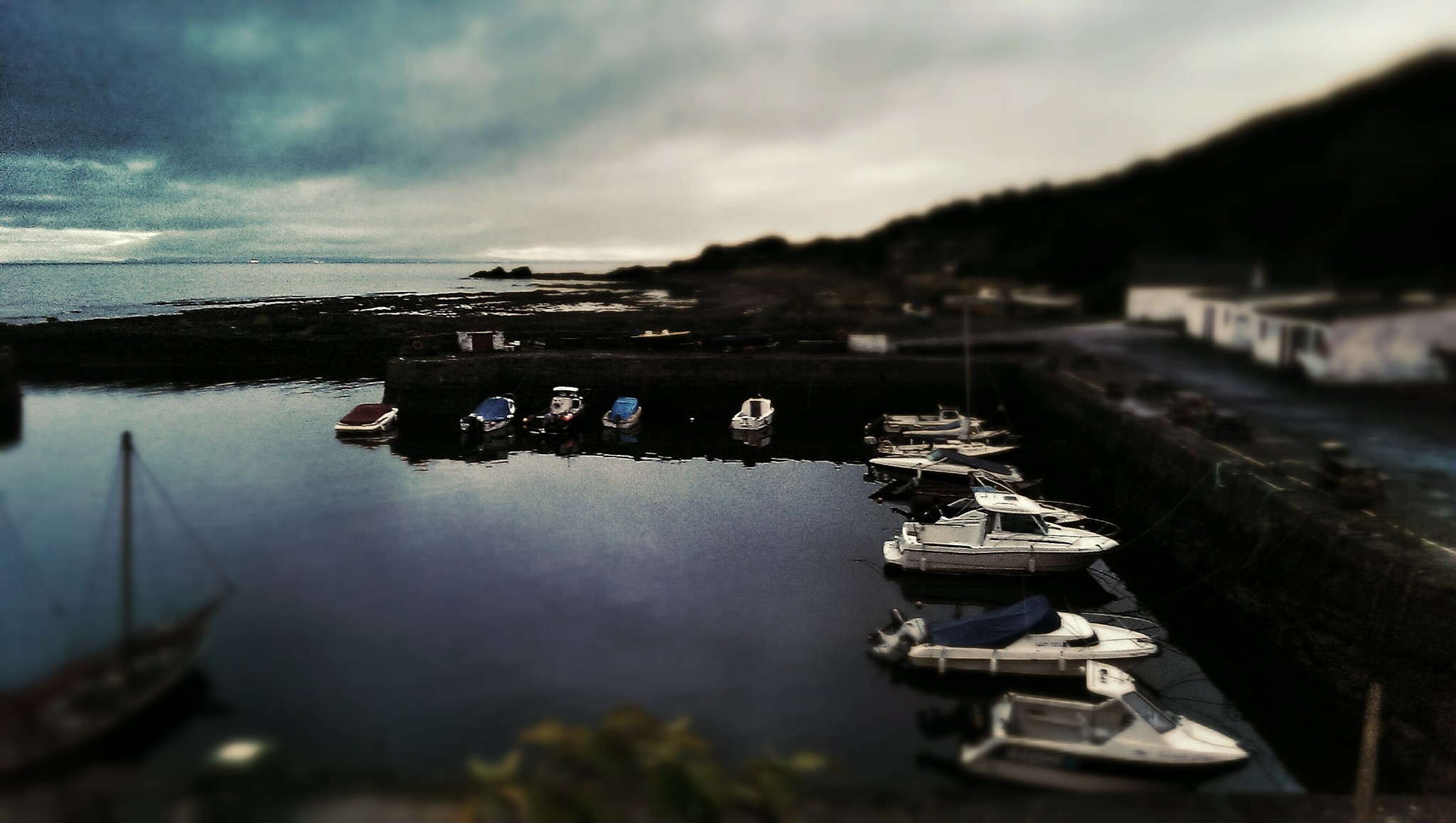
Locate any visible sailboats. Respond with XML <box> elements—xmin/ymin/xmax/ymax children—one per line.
<box><xmin>0</xmin><ymin>430</ymin><xmax>230</xmax><ymax>780</ymax></box>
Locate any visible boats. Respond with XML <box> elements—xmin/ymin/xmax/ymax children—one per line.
<box><xmin>523</xmin><ymin>386</ymin><xmax>585</xmax><ymax>435</ymax></box>
<box><xmin>699</xmin><ymin>333</ymin><xmax>780</xmax><ymax>353</ymax></box>
<box><xmin>630</xmin><ymin>330</ymin><xmax>691</xmax><ymax>345</ymax></box>
<box><xmin>882</xmin><ymin>469</ymin><xmax>1120</xmax><ymax>578</ymax></box>
<box><xmin>920</xmin><ymin>597</ymin><xmax>1253</xmax><ymax>795</ymax></box>
<box><xmin>459</xmin><ymin>393</ymin><xmax>516</xmax><ymax>433</ymax></box>
<box><xmin>865</xmin><ymin>593</ymin><xmax>1162</xmax><ymax>697</ymax></box>
<box><xmin>334</xmin><ymin>404</ymin><xmax>399</xmax><ymax>433</ymax></box>
<box><xmin>862</xmin><ymin>404</ymin><xmax>1044</xmax><ymax>502</ymax></box>
<box><xmin>798</xmin><ymin>340</ymin><xmax>847</xmax><ymax>354</ymax></box>
<box><xmin>730</xmin><ymin>394</ymin><xmax>775</xmax><ymax>431</ymax></box>
<box><xmin>602</xmin><ymin>396</ymin><xmax>642</xmax><ymax>428</ymax></box>
